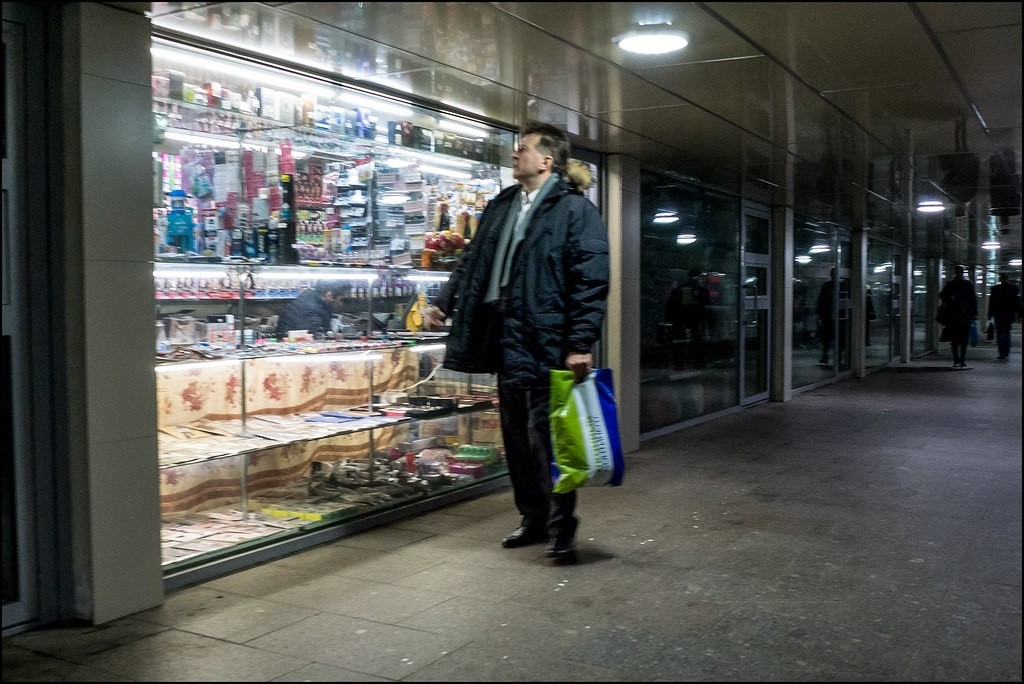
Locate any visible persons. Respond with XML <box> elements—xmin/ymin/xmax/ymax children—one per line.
<box><xmin>277</xmin><ymin>280</ymin><xmax>349</xmax><ymax>334</ymax></box>
<box><xmin>988</xmin><ymin>273</ymin><xmax>1021</xmax><ymax>358</ymax></box>
<box><xmin>940</xmin><ymin>266</ymin><xmax>978</xmax><ymax>367</ymax></box>
<box><xmin>426</xmin><ymin>125</ymin><xmax>608</xmax><ymax>555</ymax></box>
<box><xmin>817</xmin><ymin>267</ymin><xmax>836</xmax><ymax>363</ymax></box>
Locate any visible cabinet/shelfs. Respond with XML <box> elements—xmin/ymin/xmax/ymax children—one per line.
<box><xmin>147</xmin><ymin>259</ymin><xmax>505</xmax><ymax>575</ymax></box>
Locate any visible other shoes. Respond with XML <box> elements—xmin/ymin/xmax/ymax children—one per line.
<box><xmin>954</xmin><ymin>361</ymin><xmax>960</xmax><ymax>366</ymax></box>
<box><xmin>997</xmin><ymin>352</ymin><xmax>1010</xmax><ymax>359</ymax></box>
<box><xmin>962</xmin><ymin>364</ymin><xmax>966</xmax><ymax>368</ymax></box>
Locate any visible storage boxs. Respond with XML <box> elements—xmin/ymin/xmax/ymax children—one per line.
<box><xmin>221</xmin><ymin>88</ymin><xmax>232</xmax><ymax>110</ymax></box>
<box><xmin>198</xmin><ymin>83</ymin><xmax>222</xmax><ymax>108</ymax></box>
<box><xmin>448</xmin><ymin>460</ymin><xmax>487</xmax><ymax>480</ymax></box>
<box><xmin>274</xmin><ymin>90</ymin><xmax>300</xmax><ymax>126</ymax></box>
<box><xmin>453</xmin><ymin>442</ymin><xmax>500</xmax><ymax>465</ymax></box>
<box><xmin>183</xmin><ymin>83</ymin><xmax>208</xmax><ymax>106</ymax></box>
<box><xmin>302</xmin><ymin>92</ymin><xmax>379</xmax><ymax>140</ymax></box>
<box><xmin>164</xmin><ymin>314</ymin><xmax>196</xmax><ymax>345</ymax></box>
<box><xmin>255</xmin><ymin>86</ymin><xmax>275</xmax><ymax>119</ymax></box>
<box><xmin>198</xmin><ymin>322</ymin><xmax>236</xmax><ymax>347</ymax></box>
<box><xmin>156</xmin><ymin>70</ymin><xmax>186</xmax><ymax>98</ymax></box>
<box><xmin>387</xmin><ymin>121</ymin><xmax>508</xmax><ymax>164</ymax></box>
<box><xmin>150</xmin><ymin>75</ymin><xmax>170</xmax><ymax>98</ymax></box>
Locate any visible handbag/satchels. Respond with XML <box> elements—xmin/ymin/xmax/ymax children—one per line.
<box><xmin>549</xmin><ymin>368</ymin><xmax>625</xmax><ymax>494</ymax></box>
<box><xmin>987</xmin><ymin>322</ymin><xmax>994</xmax><ymax>340</ymax></box>
<box><xmin>969</xmin><ymin>322</ymin><xmax>977</xmax><ymax>346</ymax></box>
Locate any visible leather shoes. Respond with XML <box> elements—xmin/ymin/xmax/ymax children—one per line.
<box><xmin>544</xmin><ymin>540</ymin><xmax>571</xmax><ymax>553</ymax></box>
<box><xmin>502</xmin><ymin>524</ymin><xmax>550</xmax><ymax>547</ymax></box>
